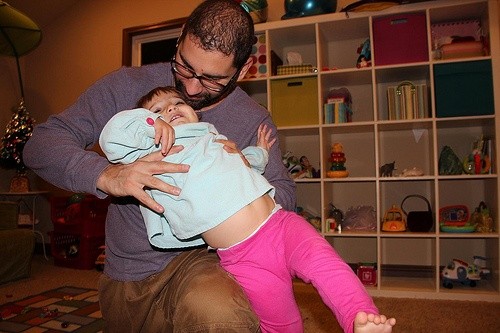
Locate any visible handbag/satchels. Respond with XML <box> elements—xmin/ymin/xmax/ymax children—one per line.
<box><xmin>387</xmin><ymin>80</ymin><xmax>428</xmax><ymax>121</ymax></box>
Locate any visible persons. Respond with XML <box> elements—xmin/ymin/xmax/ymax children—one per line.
<box><xmin>23</xmin><ymin>0</ymin><xmax>297</xmax><ymax>333</ymax></box>
<box><xmin>98</xmin><ymin>87</ymin><xmax>396</xmax><ymax>333</ymax></box>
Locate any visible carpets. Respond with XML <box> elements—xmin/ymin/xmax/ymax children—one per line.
<box><xmin>0</xmin><ymin>285</ymin><xmax>103</xmax><ymax>333</ymax></box>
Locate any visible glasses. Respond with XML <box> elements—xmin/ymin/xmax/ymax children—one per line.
<box><xmin>170</xmin><ymin>33</ymin><xmax>241</xmax><ymax>92</ymax></box>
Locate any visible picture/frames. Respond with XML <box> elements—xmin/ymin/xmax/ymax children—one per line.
<box><xmin>121</xmin><ymin>17</ymin><xmax>188</xmax><ymax>67</ymax></box>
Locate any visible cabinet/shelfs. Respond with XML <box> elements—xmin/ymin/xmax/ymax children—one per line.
<box><xmin>237</xmin><ymin>0</ymin><xmax>500</xmax><ymax>299</ymax></box>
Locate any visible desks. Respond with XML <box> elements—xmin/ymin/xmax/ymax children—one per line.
<box><xmin>0</xmin><ymin>190</ymin><xmax>50</xmax><ymax>261</ymax></box>
